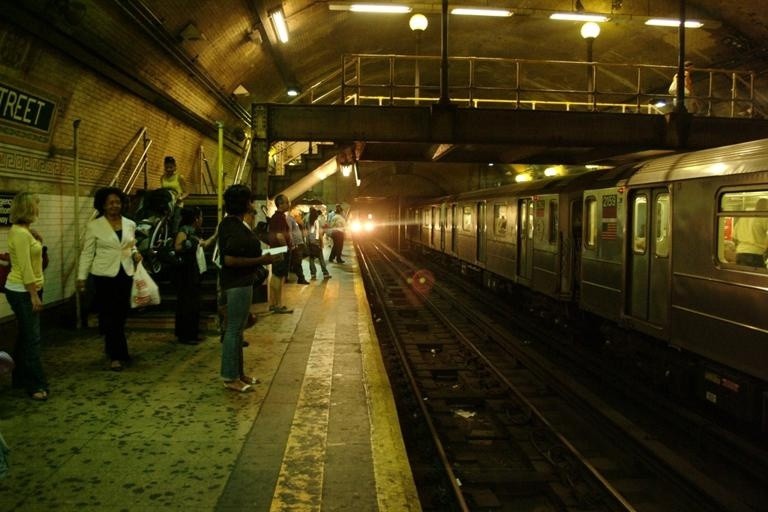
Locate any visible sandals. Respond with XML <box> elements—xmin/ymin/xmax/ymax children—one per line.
<box><xmin>224</xmin><ymin>376</ymin><xmax>261</xmax><ymax>393</ymax></box>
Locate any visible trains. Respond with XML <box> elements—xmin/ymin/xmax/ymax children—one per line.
<box><xmin>380</xmin><ymin>136</ymin><xmax>768</xmax><ymax>447</ymax></box>
<box><xmin>345</xmin><ymin>204</ymin><xmax>380</xmax><ymax>241</ymax></box>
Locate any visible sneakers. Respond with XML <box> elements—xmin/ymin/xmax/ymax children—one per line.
<box><xmin>31</xmin><ymin>389</ymin><xmax>48</xmax><ymax>400</ymax></box>
<box><xmin>269</xmin><ymin>305</ymin><xmax>293</xmax><ymax>314</ymax></box>
<box><xmin>111</xmin><ymin>360</ymin><xmax>123</xmax><ymax>371</ymax></box>
<box><xmin>178</xmin><ymin>335</ymin><xmax>203</xmax><ymax>345</ymax></box>
<box><xmin>297</xmin><ymin>258</ymin><xmax>345</xmax><ymax>284</ymax></box>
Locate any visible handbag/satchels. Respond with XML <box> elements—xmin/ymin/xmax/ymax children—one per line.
<box><xmin>253</xmin><ymin>221</ymin><xmax>270</xmax><ymax>244</ymax></box>
<box><xmin>155</xmin><ymin>245</ymin><xmax>186</xmax><ymax>268</ymax></box>
<box><xmin>310</xmin><ymin>238</ymin><xmax>322</xmax><ymax>257</ymax></box>
<box><xmin>1</xmin><ymin>246</ymin><xmax>49</xmax><ymax>294</ymax></box>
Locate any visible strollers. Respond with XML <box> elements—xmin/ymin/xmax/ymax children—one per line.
<box><xmin>129</xmin><ymin>186</ymin><xmax>179</xmax><ymax>275</ymax></box>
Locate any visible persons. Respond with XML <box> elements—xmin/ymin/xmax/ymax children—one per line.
<box><xmin>215</xmin><ymin>184</ymin><xmax>284</xmax><ymax>393</ymax></box>
<box><xmin>265</xmin><ymin>193</ymin><xmax>295</xmax><ymax>315</ymax></box>
<box><xmin>245</xmin><ymin>199</ymin><xmax>348</xmax><ymax>285</ymax></box>
<box><xmin>215</xmin><ymin>204</ymin><xmax>258</xmax><ymax>348</ymax></box>
<box><xmin>730</xmin><ymin>198</ymin><xmax>768</xmax><ymax>270</ymax></box>
<box><xmin>159</xmin><ymin>155</ymin><xmax>191</xmax><ymax>236</ymax></box>
<box><xmin>172</xmin><ymin>206</ymin><xmax>221</xmax><ymax>345</ymax></box>
<box><xmin>4</xmin><ymin>190</ymin><xmax>52</xmax><ymax>401</ymax></box>
<box><xmin>75</xmin><ymin>186</ymin><xmax>142</xmax><ymax>372</ymax></box>
